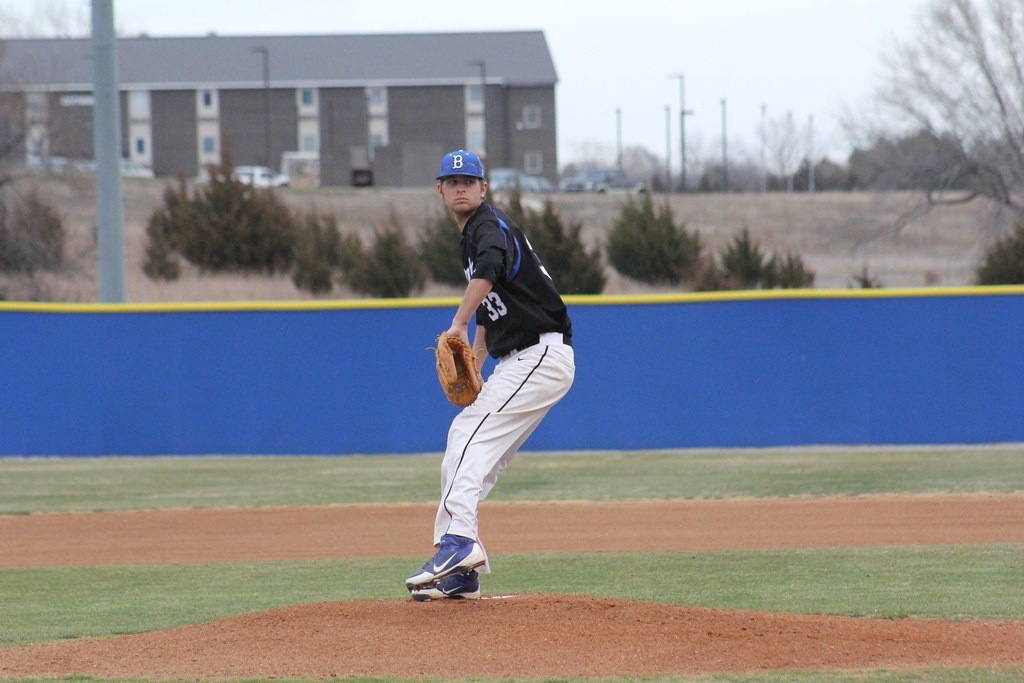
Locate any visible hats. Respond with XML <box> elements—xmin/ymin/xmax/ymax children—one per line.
<box><xmin>436</xmin><ymin>150</ymin><xmax>484</xmax><ymax>180</ymax></box>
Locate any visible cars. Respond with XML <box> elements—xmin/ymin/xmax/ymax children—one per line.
<box><xmin>485</xmin><ymin>167</ymin><xmax>552</xmax><ymax>199</ymax></box>
<box><xmin>31</xmin><ymin>151</ymin><xmax>157</xmax><ymax>179</ymax></box>
<box><xmin>229</xmin><ymin>164</ymin><xmax>292</xmax><ymax>193</ymax></box>
<box><xmin>557</xmin><ymin>165</ymin><xmax>648</xmax><ymax>196</ymax></box>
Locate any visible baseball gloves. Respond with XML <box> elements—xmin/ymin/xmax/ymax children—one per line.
<box><xmin>434</xmin><ymin>330</ymin><xmax>483</xmax><ymax>408</ymax></box>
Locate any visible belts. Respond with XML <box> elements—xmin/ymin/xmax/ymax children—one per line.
<box><xmin>510</xmin><ymin>333</ymin><xmax>573</xmax><ymax>356</ymax></box>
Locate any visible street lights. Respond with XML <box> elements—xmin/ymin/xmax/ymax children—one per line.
<box><xmin>253</xmin><ymin>45</ymin><xmax>270</xmax><ymax>167</ymax></box>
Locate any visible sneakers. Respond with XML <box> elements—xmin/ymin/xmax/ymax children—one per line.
<box><xmin>411</xmin><ymin>570</ymin><xmax>481</xmax><ymax>602</ymax></box>
<box><xmin>405</xmin><ymin>535</ymin><xmax>486</xmax><ymax>591</ymax></box>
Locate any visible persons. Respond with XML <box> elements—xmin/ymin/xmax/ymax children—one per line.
<box><xmin>406</xmin><ymin>150</ymin><xmax>575</xmax><ymax>602</ymax></box>
